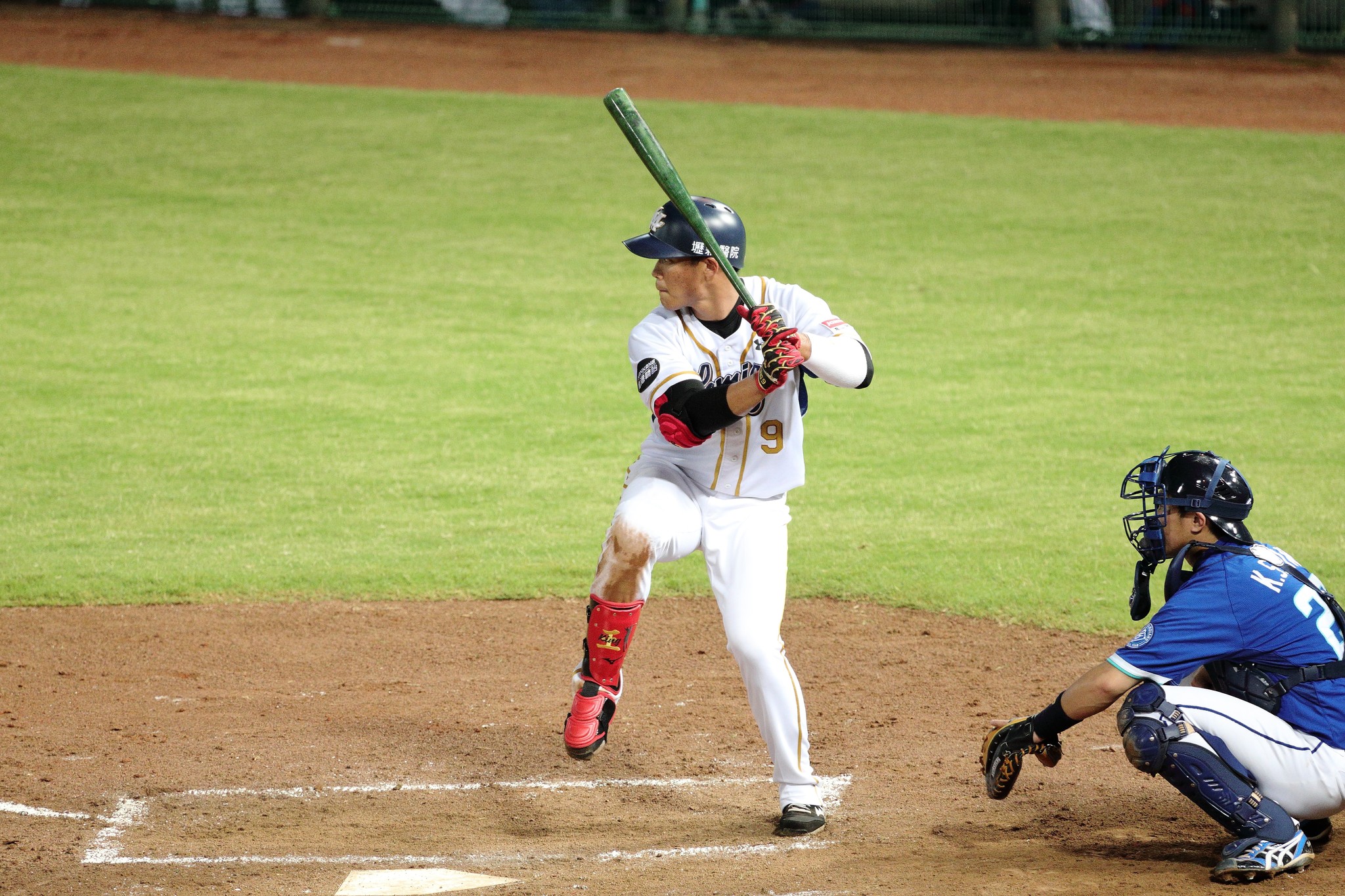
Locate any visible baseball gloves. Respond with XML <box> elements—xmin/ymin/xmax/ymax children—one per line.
<box><xmin>978</xmin><ymin>714</ymin><xmax>1063</xmax><ymax>801</ymax></box>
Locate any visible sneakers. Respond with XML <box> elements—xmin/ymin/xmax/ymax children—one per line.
<box><xmin>564</xmin><ymin>668</ymin><xmax>623</xmax><ymax>760</ymax></box>
<box><xmin>775</xmin><ymin>803</ymin><xmax>827</xmax><ymax>836</ymax></box>
<box><xmin>1214</xmin><ymin>824</ymin><xmax>1315</xmax><ymax>883</ymax></box>
<box><xmin>1299</xmin><ymin>817</ymin><xmax>1332</xmax><ymax>843</ymax></box>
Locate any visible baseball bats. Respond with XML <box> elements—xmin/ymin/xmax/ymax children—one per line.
<box><xmin>602</xmin><ymin>84</ymin><xmax>797</xmax><ymax>377</ymax></box>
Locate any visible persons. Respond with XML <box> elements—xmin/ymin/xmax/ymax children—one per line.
<box><xmin>184</xmin><ymin>1</ymin><xmax>1345</xmax><ymax>89</ymax></box>
<box><xmin>977</xmin><ymin>446</ymin><xmax>1345</xmax><ymax>886</ymax></box>
<box><xmin>562</xmin><ymin>196</ymin><xmax>874</xmax><ymax>837</ymax></box>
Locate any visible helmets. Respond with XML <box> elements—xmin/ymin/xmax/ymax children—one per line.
<box><xmin>621</xmin><ymin>196</ymin><xmax>746</xmax><ymax>269</ymax></box>
<box><xmin>1161</xmin><ymin>452</ymin><xmax>1254</xmax><ymax>545</ymax></box>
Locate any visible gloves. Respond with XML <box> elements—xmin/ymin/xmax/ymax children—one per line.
<box><xmin>736</xmin><ymin>303</ymin><xmax>787</xmax><ymax>342</ymax></box>
<box><xmin>756</xmin><ymin>327</ymin><xmax>804</xmax><ymax>395</ymax></box>
